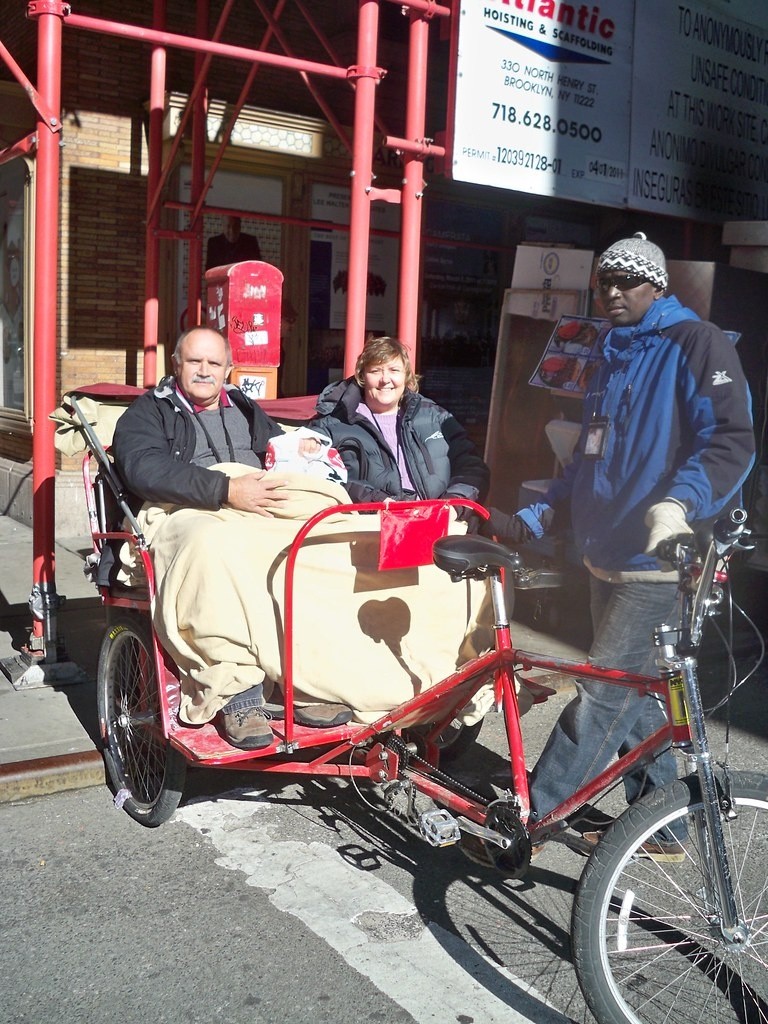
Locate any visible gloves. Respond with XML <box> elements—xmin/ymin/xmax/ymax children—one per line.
<box><xmin>644</xmin><ymin>498</ymin><xmax>695</xmax><ymax>574</ymax></box>
<box><xmin>467</xmin><ymin>505</ymin><xmax>530</xmax><ymax>545</ymax></box>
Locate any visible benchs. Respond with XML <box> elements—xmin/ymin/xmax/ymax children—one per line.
<box><xmin>45</xmin><ymin>382</ymin><xmax>490</xmax><ymax>613</ymax></box>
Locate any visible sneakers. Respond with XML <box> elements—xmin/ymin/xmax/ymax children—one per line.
<box><xmin>293</xmin><ymin>701</ymin><xmax>350</xmax><ymax>726</ymax></box>
<box><xmin>221</xmin><ymin>706</ymin><xmax>271</xmax><ymax>749</ymax></box>
<box><xmin>577</xmin><ymin>828</ymin><xmax>688</xmax><ymax>865</ymax></box>
<box><xmin>466</xmin><ymin>839</ymin><xmax>544</xmax><ymax>866</ymax></box>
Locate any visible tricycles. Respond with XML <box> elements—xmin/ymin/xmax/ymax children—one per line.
<box><xmin>41</xmin><ymin>380</ymin><xmax>768</xmax><ymax>1024</ymax></box>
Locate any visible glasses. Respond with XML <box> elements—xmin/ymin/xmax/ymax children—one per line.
<box><xmin>597</xmin><ymin>272</ymin><xmax>649</xmax><ymax>294</ymax></box>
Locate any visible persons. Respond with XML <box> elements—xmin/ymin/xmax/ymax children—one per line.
<box><xmin>453</xmin><ymin>232</ymin><xmax>755</xmax><ymax>870</ymax></box>
<box><xmin>309</xmin><ymin>334</ymin><xmax>489</xmax><ymax>519</ymax></box>
<box><xmin>114</xmin><ymin>326</ymin><xmax>354</xmax><ymax>750</ymax></box>
<box><xmin>206</xmin><ymin>215</ymin><xmax>261</xmax><ymax>271</ymax></box>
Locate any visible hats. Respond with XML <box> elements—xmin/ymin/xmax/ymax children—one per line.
<box><xmin>595</xmin><ymin>233</ymin><xmax>668</xmax><ymax>291</ymax></box>
